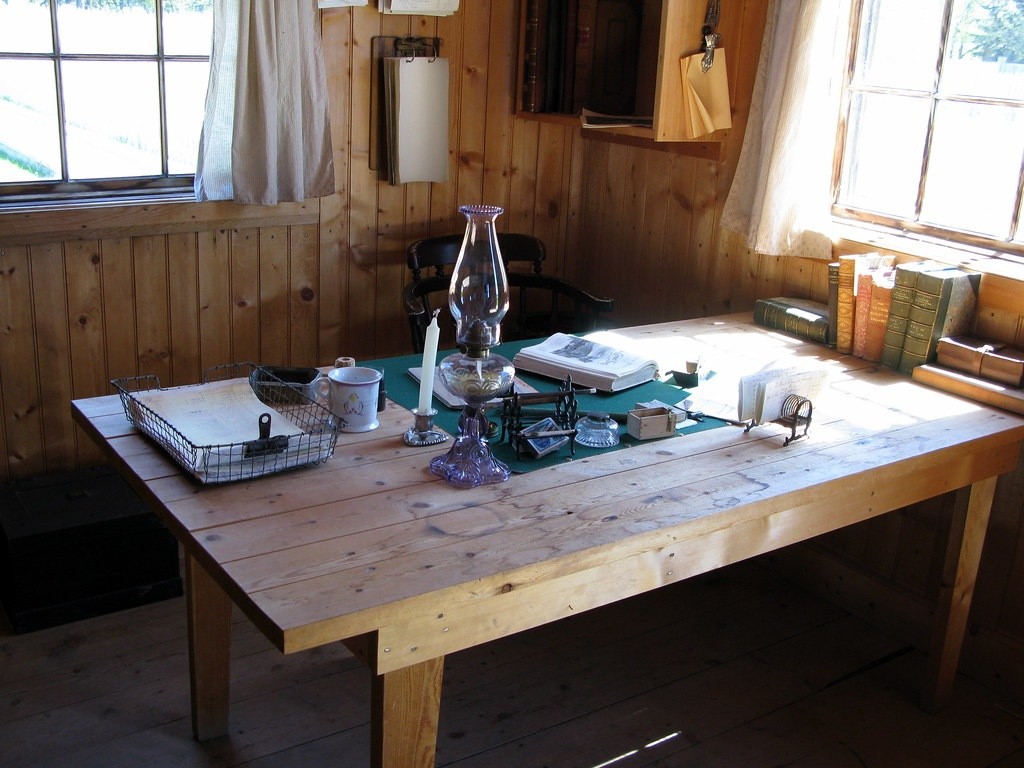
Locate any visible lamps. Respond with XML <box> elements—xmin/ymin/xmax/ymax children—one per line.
<box><xmin>427</xmin><ymin>202</ymin><xmax>518</xmax><ymax>490</ymax></box>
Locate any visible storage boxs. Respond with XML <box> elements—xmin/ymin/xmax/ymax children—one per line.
<box><xmin>0</xmin><ymin>460</ymin><xmax>183</xmax><ymax>638</ymax></box>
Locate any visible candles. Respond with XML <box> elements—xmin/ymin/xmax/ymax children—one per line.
<box><xmin>411</xmin><ymin>307</ymin><xmax>444</xmax><ymax>414</ymax></box>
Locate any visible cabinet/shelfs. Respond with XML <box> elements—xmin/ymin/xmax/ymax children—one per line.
<box><xmin>508</xmin><ymin>0</ymin><xmax>710</xmax><ymax>143</ymax></box>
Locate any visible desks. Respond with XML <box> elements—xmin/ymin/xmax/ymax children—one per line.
<box><xmin>70</xmin><ymin>301</ymin><xmax>1024</xmax><ymax>768</ymax></box>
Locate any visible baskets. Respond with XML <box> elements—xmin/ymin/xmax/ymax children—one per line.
<box><xmin>109</xmin><ymin>362</ymin><xmax>351</xmax><ymax>484</ymax></box>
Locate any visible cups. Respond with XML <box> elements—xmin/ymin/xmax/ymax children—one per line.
<box><xmin>327</xmin><ymin>366</ymin><xmax>383</xmax><ymax>434</ymax></box>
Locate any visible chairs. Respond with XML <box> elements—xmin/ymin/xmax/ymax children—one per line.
<box><xmin>398</xmin><ymin>229</ymin><xmax>616</xmax><ymax>351</ymax></box>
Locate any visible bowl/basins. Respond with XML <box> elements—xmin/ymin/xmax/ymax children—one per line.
<box><xmin>249</xmin><ymin>364</ymin><xmax>322</xmax><ymax>405</ymax></box>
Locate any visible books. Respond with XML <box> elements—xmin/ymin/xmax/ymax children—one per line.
<box><xmin>753</xmin><ymin>252</ymin><xmax>1024</xmax><ymax>415</ymax></box>
<box><xmin>512</xmin><ymin>332</ymin><xmax>659</xmax><ymax>393</ymax></box>
<box><xmin>407</xmin><ymin>367</ymin><xmax>538</xmax><ymax>409</ymax></box>
